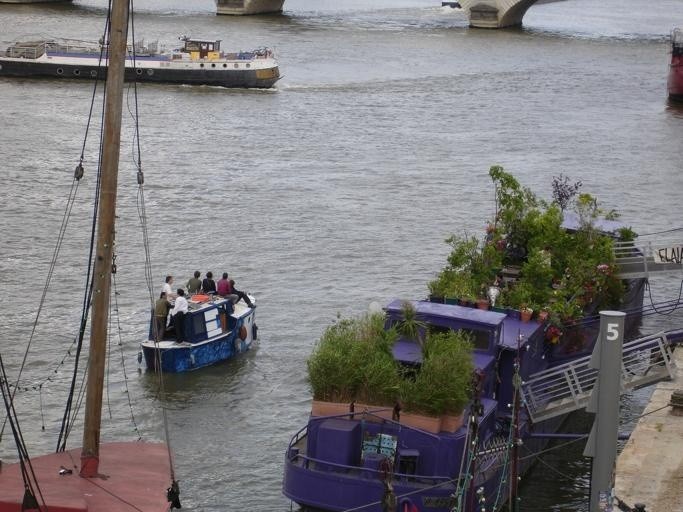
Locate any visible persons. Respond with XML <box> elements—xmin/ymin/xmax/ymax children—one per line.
<box><xmin>151</xmin><ymin>292</ymin><xmax>174</xmax><ymax>342</ymax></box>
<box><xmin>172</xmin><ymin>288</ymin><xmax>188</xmax><ymax>343</ymax></box>
<box><xmin>185</xmin><ymin>271</ymin><xmax>256</xmax><ymax>311</ymax></box>
<box><xmin>161</xmin><ymin>275</ymin><xmax>178</xmax><ymax>302</ymax></box>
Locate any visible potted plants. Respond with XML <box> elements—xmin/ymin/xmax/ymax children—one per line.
<box><xmin>425</xmin><ymin>261</ymin><xmax>537</xmax><ymax>321</ymax></box>
<box><xmin>305</xmin><ymin>311</ymin><xmax>474</xmax><ymax>436</ymax></box>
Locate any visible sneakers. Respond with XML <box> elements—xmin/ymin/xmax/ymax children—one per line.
<box><xmin>248</xmin><ymin>304</ymin><xmax>256</xmax><ymax>308</ymax></box>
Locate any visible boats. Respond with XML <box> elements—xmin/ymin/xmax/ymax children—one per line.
<box><xmin>665</xmin><ymin>27</ymin><xmax>683</xmax><ymax>103</ymax></box>
<box><xmin>276</xmin><ymin>203</ymin><xmax>652</xmax><ymax>512</ymax></box>
<box><xmin>140</xmin><ymin>290</ymin><xmax>259</xmax><ymax>376</ymax></box>
<box><xmin>0</xmin><ymin>36</ymin><xmax>284</xmax><ymax>90</ymax></box>
